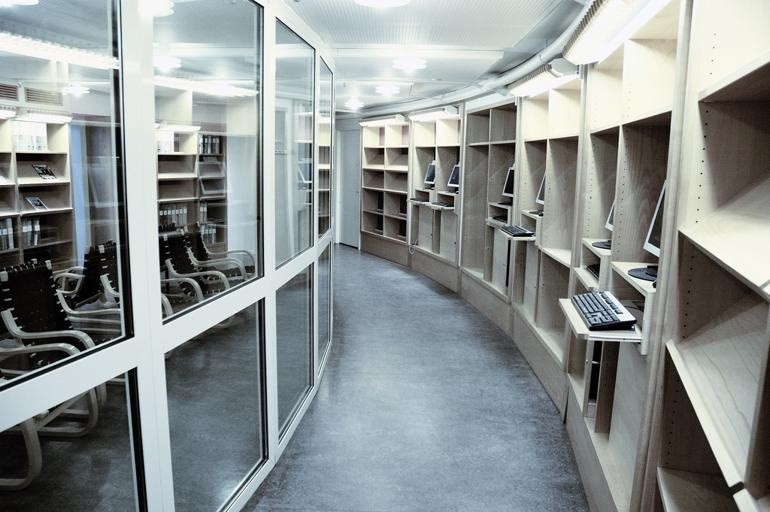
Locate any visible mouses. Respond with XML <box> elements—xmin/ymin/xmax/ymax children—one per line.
<box><xmin>538</xmin><ymin>212</ymin><xmax>543</xmax><ymax>216</ymax></box>
<box><xmin>456</xmin><ymin>191</ymin><xmax>459</xmax><ymax>194</ymax></box>
<box><xmin>430</xmin><ymin>188</ymin><xmax>435</xmax><ymax>190</ymax></box>
<box><xmin>652</xmin><ymin>279</ymin><xmax>657</xmax><ymax>288</ymax></box>
<box><xmin>302</xmin><ymin>186</ymin><xmax>306</xmax><ymax>191</ymax></box>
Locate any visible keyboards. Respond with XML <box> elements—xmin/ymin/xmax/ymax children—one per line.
<box><xmin>433</xmin><ymin>201</ymin><xmax>454</xmax><ymax>207</ymax></box>
<box><xmin>571</xmin><ymin>290</ymin><xmax>637</xmax><ymax>331</ymax></box>
<box><xmin>493</xmin><ymin>215</ymin><xmax>507</xmax><ymax>223</ymax></box>
<box><xmin>586</xmin><ymin>264</ymin><xmax>600</xmax><ymax>279</ymax></box>
<box><xmin>499</xmin><ymin>225</ymin><xmax>534</xmax><ymax>236</ymax></box>
<box><xmin>410</xmin><ymin>197</ymin><xmax>429</xmax><ymax>201</ymax></box>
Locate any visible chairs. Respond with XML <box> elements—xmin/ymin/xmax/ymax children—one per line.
<box><xmin>96</xmin><ymin>242</ymin><xmax>205</xmax><ymax>358</ymax></box>
<box><xmin>165</xmin><ymin>232</ymin><xmax>247</xmax><ymax>327</ymax></box>
<box><xmin>184</xmin><ymin>231</ymin><xmax>256</xmax><ymax>279</ymax></box>
<box><xmin>0</xmin><ymin>344</ymin><xmax>98</xmax><ymax>492</ymax></box>
<box><xmin>1</xmin><ymin>259</ymin><xmax>125</xmax><ymax>419</ymax></box>
<box><xmin>53</xmin><ymin>245</ymin><xmax>100</xmax><ymax>309</ymax></box>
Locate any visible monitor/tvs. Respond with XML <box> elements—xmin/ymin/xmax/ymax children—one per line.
<box><xmin>298</xmin><ymin>161</ymin><xmax>313</xmax><ymax>184</ymax></box>
<box><xmin>447</xmin><ymin>165</ymin><xmax>459</xmax><ymax>193</ymax></box>
<box><xmin>498</xmin><ymin>168</ymin><xmax>515</xmax><ymax>206</ymax></box>
<box><xmin>424</xmin><ymin>164</ymin><xmax>435</xmax><ymax>190</ymax></box>
<box><xmin>89</xmin><ymin>165</ymin><xmax>119</xmax><ymax>208</ymax></box>
<box><xmin>530</xmin><ymin>169</ymin><xmax>546</xmax><ymax>216</ymax></box>
<box><xmin>628</xmin><ymin>180</ymin><xmax>666</xmax><ymax>281</ymax></box>
<box><xmin>591</xmin><ymin>197</ymin><xmax>615</xmax><ymax>249</ymax></box>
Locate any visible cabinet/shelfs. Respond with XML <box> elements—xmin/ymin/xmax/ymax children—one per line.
<box><xmin>566</xmin><ymin>0</ymin><xmax>693</xmax><ymax>510</ymax></box>
<box><xmin>410</xmin><ymin>102</ymin><xmax>465</xmax><ymax>296</ymax></box>
<box><xmin>360</xmin><ymin>120</ymin><xmax>410</xmax><ymax>269</ymax></box>
<box><xmin>512</xmin><ymin>67</ymin><xmax>589</xmax><ymax>409</ymax></box>
<box><xmin>459</xmin><ymin>95</ymin><xmax>523</xmax><ymax>338</ymax></box>
<box><xmin>0</xmin><ymin>111</ymin><xmax>229</xmax><ymax>269</ymax></box>
<box><xmin>632</xmin><ymin>0</ymin><xmax>770</xmax><ymax>512</ymax></box>
